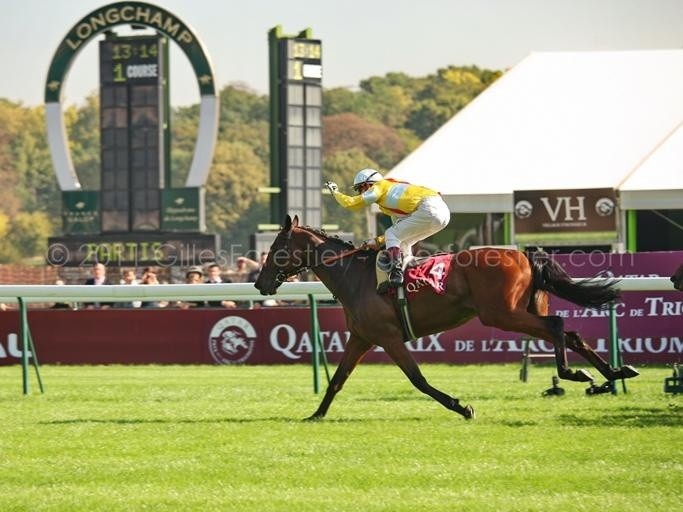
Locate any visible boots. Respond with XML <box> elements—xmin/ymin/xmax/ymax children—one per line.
<box><xmin>377</xmin><ymin>247</ymin><xmax>404</xmax><ymax>294</ymax></box>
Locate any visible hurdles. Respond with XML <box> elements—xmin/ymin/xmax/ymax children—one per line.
<box><xmin>0</xmin><ymin>282</ymin><xmax>334</xmax><ymax>395</ymax></box>
<box><xmin>519</xmin><ymin>278</ymin><xmax>676</xmax><ymax>394</ymax></box>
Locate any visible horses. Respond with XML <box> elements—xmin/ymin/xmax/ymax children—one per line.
<box><xmin>669</xmin><ymin>263</ymin><xmax>683</xmax><ymax>292</ymax></box>
<box><xmin>255</xmin><ymin>214</ymin><xmax>640</xmax><ymax>421</ymax></box>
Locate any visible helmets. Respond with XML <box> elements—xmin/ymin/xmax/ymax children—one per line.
<box><xmin>354</xmin><ymin>169</ymin><xmax>383</xmax><ymax>191</ymax></box>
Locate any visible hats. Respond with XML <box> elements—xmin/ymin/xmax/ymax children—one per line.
<box><xmin>186</xmin><ymin>266</ymin><xmax>203</xmax><ymax>279</ymax></box>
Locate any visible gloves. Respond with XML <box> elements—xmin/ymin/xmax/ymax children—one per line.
<box><xmin>327</xmin><ymin>181</ymin><xmax>338</xmax><ymax>195</ymax></box>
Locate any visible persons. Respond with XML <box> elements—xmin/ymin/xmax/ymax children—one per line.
<box><xmin>50</xmin><ymin>253</ymin><xmax>319</xmax><ymax>308</ymax></box>
<box><xmin>324</xmin><ymin>169</ymin><xmax>451</xmax><ymax>292</ymax></box>
